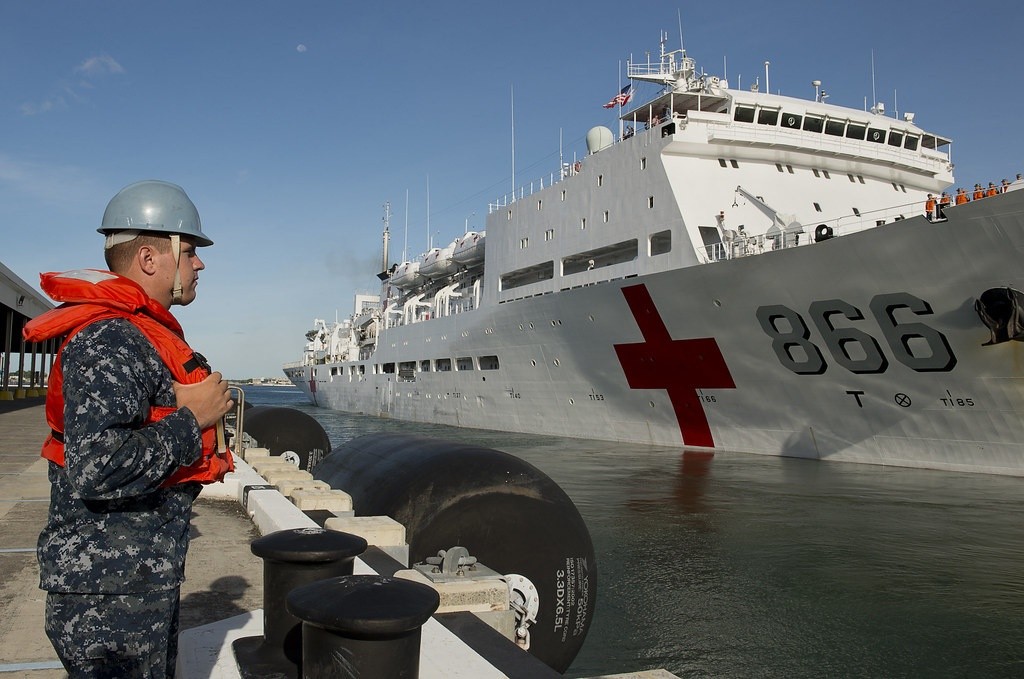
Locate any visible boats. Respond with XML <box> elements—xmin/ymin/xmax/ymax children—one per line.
<box><xmin>419</xmin><ymin>240</ymin><xmax>458</xmax><ymax>280</ymax></box>
<box><xmin>388</xmin><ymin>260</ymin><xmax>430</xmax><ymax>290</ymax></box>
<box><xmin>450</xmin><ymin>230</ymin><xmax>487</xmax><ymax>267</ymax></box>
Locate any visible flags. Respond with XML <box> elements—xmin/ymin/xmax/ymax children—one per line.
<box><xmin>602</xmin><ymin>84</ymin><xmax>632</xmax><ymax>108</ymax></box>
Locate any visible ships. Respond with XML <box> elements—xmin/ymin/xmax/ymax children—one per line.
<box><xmin>283</xmin><ymin>6</ymin><xmax>1024</xmax><ymax>480</ymax></box>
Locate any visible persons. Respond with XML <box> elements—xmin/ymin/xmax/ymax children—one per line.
<box><xmin>973</xmin><ymin>183</ymin><xmax>984</xmax><ymax>200</ymax></box>
<box><xmin>940</xmin><ymin>191</ymin><xmax>949</xmax><ymax>203</ymax></box>
<box><xmin>22</xmin><ymin>179</ymin><xmax>234</xmax><ymax>679</ymax></box>
<box><xmin>1016</xmin><ymin>173</ymin><xmax>1022</xmax><ymax>180</ymax></box>
<box><xmin>925</xmin><ymin>194</ymin><xmax>937</xmax><ymax>221</ymax></box>
<box><xmin>986</xmin><ymin>181</ymin><xmax>999</xmax><ymax>198</ymax></box>
<box><xmin>955</xmin><ymin>188</ymin><xmax>970</xmax><ymax>205</ymax></box>
<box><xmin>1000</xmin><ymin>179</ymin><xmax>1009</xmax><ymax>193</ymax></box>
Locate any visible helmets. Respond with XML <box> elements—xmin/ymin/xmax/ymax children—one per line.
<box><xmin>1001</xmin><ymin>179</ymin><xmax>1007</xmax><ymax>182</ymax></box>
<box><xmin>942</xmin><ymin>192</ymin><xmax>948</xmax><ymax>194</ymax></box>
<box><xmin>974</xmin><ymin>184</ymin><xmax>979</xmax><ymax>187</ymax></box>
<box><xmin>956</xmin><ymin>188</ymin><xmax>963</xmax><ymax>191</ymax></box>
<box><xmin>989</xmin><ymin>182</ymin><xmax>994</xmax><ymax>185</ymax></box>
<box><xmin>1016</xmin><ymin>174</ymin><xmax>1021</xmax><ymax>176</ymax></box>
<box><xmin>97</xmin><ymin>179</ymin><xmax>214</xmax><ymax>247</ymax></box>
<box><xmin>979</xmin><ymin>184</ymin><xmax>981</xmax><ymax>186</ymax></box>
<box><xmin>927</xmin><ymin>193</ymin><xmax>932</xmax><ymax>196</ymax></box>
